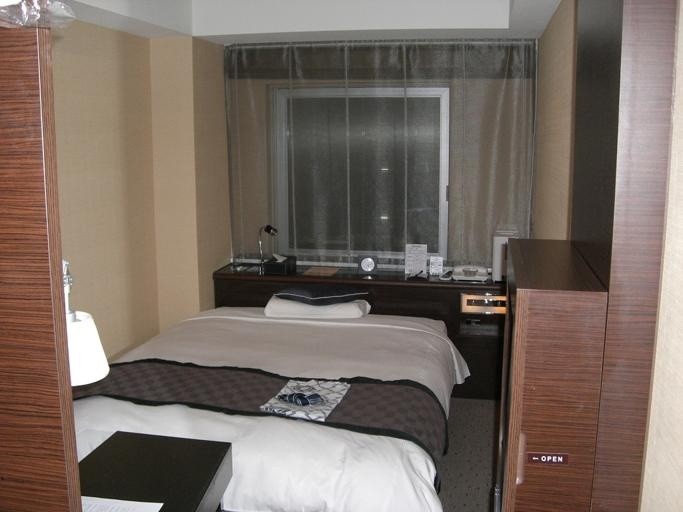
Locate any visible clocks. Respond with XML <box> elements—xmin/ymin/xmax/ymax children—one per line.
<box><xmin>357</xmin><ymin>254</ymin><xmax>379</xmax><ymax>276</ymax></box>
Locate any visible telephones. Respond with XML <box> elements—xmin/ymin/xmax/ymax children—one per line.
<box><xmin>452</xmin><ymin>265</ymin><xmax>488</xmax><ymax>281</ymax></box>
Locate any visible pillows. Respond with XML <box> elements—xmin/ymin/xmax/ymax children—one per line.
<box><xmin>272</xmin><ymin>286</ymin><xmax>369</xmax><ymax>303</ymax></box>
<box><xmin>264</xmin><ymin>294</ymin><xmax>372</xmax><ymax>317</ymax></box>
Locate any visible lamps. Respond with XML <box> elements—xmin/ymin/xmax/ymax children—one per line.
<box><xmin>62</xmin><ymin>258</ymin><xmax>110</xmax><ymax>386</ymax></box>
<box><xmin>258</xmin><ymin>225</ymin><xmax>278</xmax><ymax>264</ymax></box>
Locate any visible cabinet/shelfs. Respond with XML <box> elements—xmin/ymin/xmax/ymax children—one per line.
<box><xmin>213</xmin><ymin>261</ymin><xmax>506</xmax><ymax>400</ymax></box>
<box><xmin>486</xmin><ymin>237</ymin><xmax>609</xmax><ymax>511</ymax></box>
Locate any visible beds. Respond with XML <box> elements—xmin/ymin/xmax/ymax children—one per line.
<box><xmin>72</xmin><ymin>305</ymin><xmax>472</xmax><ymax>512</ymax></box>
